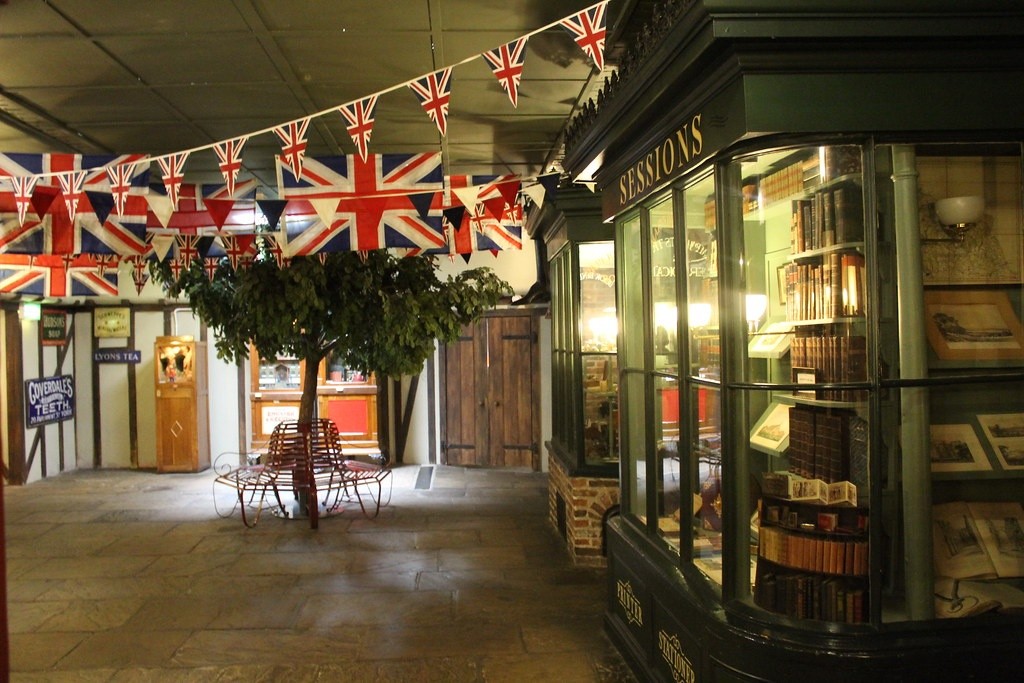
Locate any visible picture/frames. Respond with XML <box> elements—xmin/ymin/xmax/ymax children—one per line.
<box><xmin>775</xmin><ymin>265</ymin><xmax>786</xmax><ymax>305</ymax></box>
<box><xmin>925</xmin><ymin>284</ymin><xmax>1024</xmax><ymax>484</ymax></box>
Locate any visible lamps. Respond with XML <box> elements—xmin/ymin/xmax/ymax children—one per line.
<box><xmin>921</xmin><ymin>194</ymin><xmax>988</xmax><ymax>245</ymax></box>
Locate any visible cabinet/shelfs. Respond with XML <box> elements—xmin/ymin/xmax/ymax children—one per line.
<box><xmin>153</xmin><ymin>335</ymin><xmax>211</xmax><ymax>473</ymax></box>
<box><xmin>752</xmin><ymin>144</ymin><xmax>868</xmax><ymax>617</ymax></box>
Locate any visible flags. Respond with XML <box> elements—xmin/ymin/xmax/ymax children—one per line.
<box><xmin>274</xmin><ymin>151</ymin><xmax>446</xmax><ymax>258</ymax></box>
<box><xmin>0</xmin><ymin>151</ymin><xmax>151</xmax><ymax>255</ymax></box>
<box><xmin>0</xmin><ymin>253</ymin><xmax>118</xmax><ymax>298</ymax></box>
<box><xmin>141</xmin><ymin>179</ymin><xmax>259</xmax><ymax>258</ymax></box>
<box><xmin>394</xmin><ymin>174</ymin><xmax>525</xmax><ymax>257</ymax></box>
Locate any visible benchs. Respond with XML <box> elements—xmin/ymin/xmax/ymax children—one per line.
<box><xmin>213</xmin><ymin>418</ymin><xmax>391</xmax><ymax>527</ymax></box>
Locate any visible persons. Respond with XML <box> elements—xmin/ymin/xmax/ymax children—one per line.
<box><xmin>159</xmin><ymin>347</ymin><xmax>191</xmax><ymax>383</ymax></box>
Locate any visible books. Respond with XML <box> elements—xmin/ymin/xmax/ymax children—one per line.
<box><xmin>932</xmin><ymin>502</ymin><xmax>1024</xmax><ymax>618</ymax></box>
<box><xmin>759</xmin><ymin>153</ymin><xmax>822</xmax><ymax>206</ymax></box>
<box><xmin>926</xmin><ymin>288</ymin><xmax>1024</xmax><ymax>361</ymax></box>
<box><xmin>785</xmin><ymin>250</ymin><xmax>866</xmax><ymax>322</ymax></box>
<box><xmin>928</xmin><ymin>412</ymin><xmax>1024</xmax><ymax>482</ymax></box>
<box><xmin>755</xmin><ymin>528</ymin><xmax>868</xmax><ymax>623</ymax></box>
<box><xmin>791</xmin><ymin>190</ymin><xmax>884</xmax><ymax>255</ymax></box>
<box><xmin>704</xmin><ymin>185</ymin><xmax>758</xmax><ymax>232</ymax></box>
<box><xmin>790</xmin><ymin>336</ymin><xmax>866</xmax><ymax>402</ymax></box>
<box><xmin>789</xmin><ymin>406</ymin><xmax>857</xmax><ymax>485</ymax></box>
